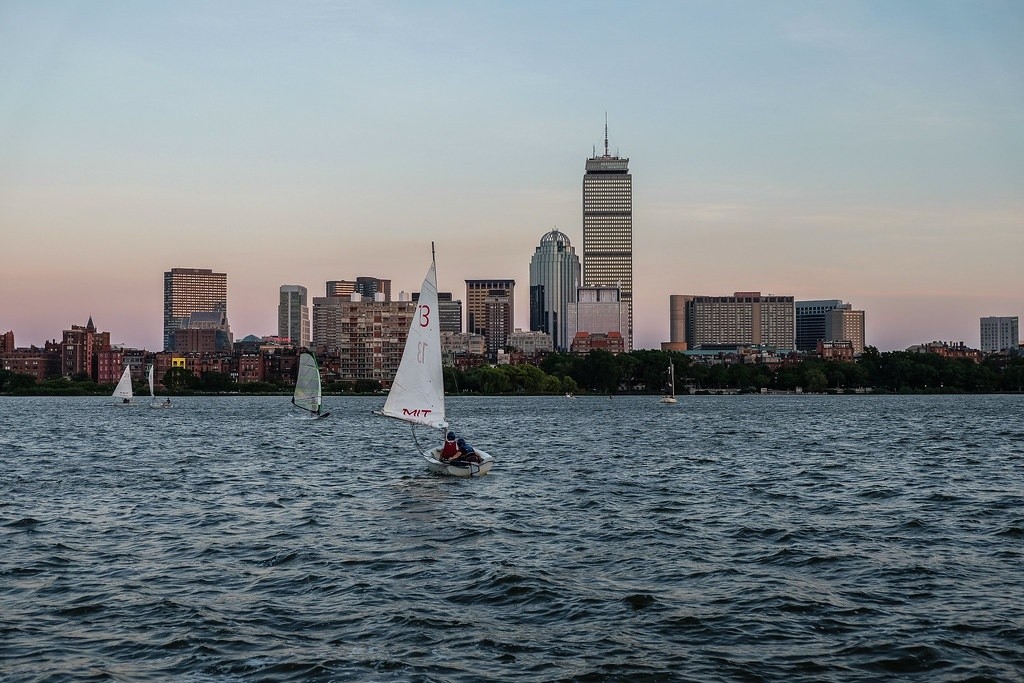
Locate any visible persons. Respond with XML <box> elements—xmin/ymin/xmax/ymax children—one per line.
<box><xmin>439</xmin><ymin>431</ymin><xmax>459</xmax><ymax>462</ymax></box>
<box><xmin>446</xmin><ymin>438</ymin><xmax>480</xmax><ymax>467</ymax></box>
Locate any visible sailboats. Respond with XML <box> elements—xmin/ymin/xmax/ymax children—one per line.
<box><xmin>147</xmin><ymin>364</ymin><xmax>173</xmax><ymax>408</ymax></box>
<box><xmin>288</xmin><ymin>347</ymin><xmax>332</xmax><ymax>423</ymax></box>
<box><xmin>111</xmin><ymin>364</ymin><xmax>133</xmax><ymax>405</ymax></box>
<box><xmin>658</xmin><ymin>358</ymin><xmax>677</xmax><ymax>404</ymax></box>
<box><xmin>374</xmin><ymin>239</ymin><xmax>492</xmax><ymax>482</ymax></box>
